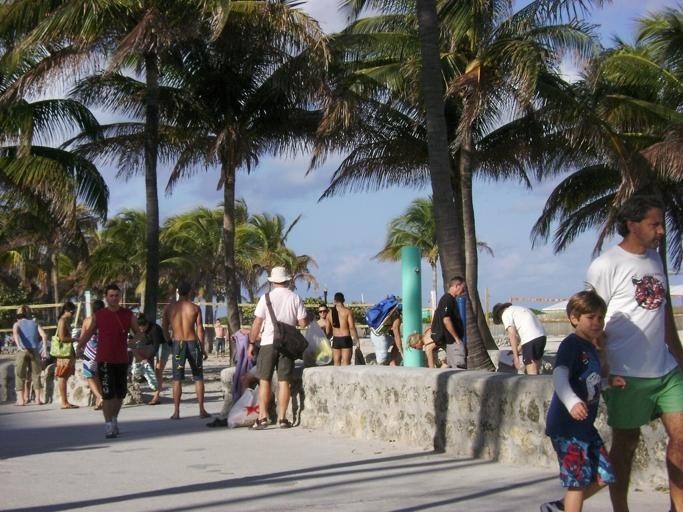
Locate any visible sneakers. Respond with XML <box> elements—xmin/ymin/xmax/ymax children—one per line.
<box><xmin>104</xmin><ymin>416</ymin><xmax>119</xmax><ymax>438</ymax></box>
<box><xmin>279</xmin><ymin>418</ymin><xmax>292</xmax><ymax>428</ymax></box>
<box><xmin>540</xmin><ymin>501</ymin><xmax>566</xmax><ymax>512</ymax></box>
<box><xmin>248</xmin><ymin>417</ymin><xmax>268</xmax><ymax>430</ymax></box>
<box><xmin>206</xmin><ymin>418</ymin><xmax>227</xmax><ymax>427</ymax></box>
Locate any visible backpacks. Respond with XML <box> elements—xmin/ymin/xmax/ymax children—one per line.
<box><xmin>362</xmin><ymin>296</ymin><xmax>399</xmax><ymax>336</ymax></box>
<box><xmin>429</xmin><ymin>310</ymin><xmax>447</xmax><ymax>352</ymax></box>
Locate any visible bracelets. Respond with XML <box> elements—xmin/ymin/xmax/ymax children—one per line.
<box><xmin>248</xmin><ymin>341</ymin><xmax>256</xmax><ymax>345</ymax></box>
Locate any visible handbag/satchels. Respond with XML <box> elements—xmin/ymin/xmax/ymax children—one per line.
<box><xmin>271</xmin><ymin>321</ymin><xmax>309</xmax><ymax>361</ymax></box>
<box><xmin>50</xmin><ymin>333</ymin><xmax>74</xmax><ymax>358</ymax></box>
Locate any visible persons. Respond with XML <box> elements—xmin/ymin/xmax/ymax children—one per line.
<box><xmin>324</xmin><ymin>292</ymin><xmax>359</xmax><ymax>365</ymax></box>
<box><xmin>431</xmin><ymin>278</ymin><xmax>470</xmax><ymax>369</ymax></box>
<box><xmin>316</xmin><ymin>303</ymin><xmax>329</xmax><ymax>336</ymax></box>
<box><xmin>203</xmin><ymin>328</ymin><xmax>258</xmax><ymax>428</ymax></box>
<box><xmin>370</xmin><ymin>303</ymin><xmax>402</xmax><ymax>364</ymax></box>
<box><xmin>409</xmin><ymin>326</ymin><xmax>448</xmax><ymax>369</ymax></box>
<box><xmin>243</xmin><ymin>265</ymin><xmax>309</xmax><ymax>429</ymax></box>
<box><xmin>12</xmin><ymin>282</ymin><xmax>211</xmax><ymax>438</ymax></box>
<box><xmin>540</xmin><ymin>293</ymin><xmax>627</xmax><ymax>512</ymax></box>
<box><xmin>212</xmin><ymin>319</ymin><xmax>227</xmax><ymax>358</ymax></box>
<box><xmin>492</xmin><ymin>303</ymin><xmax>547</xmax><ymax>376</ymax></box>
<box><xmin>587</xmin><ymin>194</ymin><xmax>681</xmax><ymax>512</ymax></box>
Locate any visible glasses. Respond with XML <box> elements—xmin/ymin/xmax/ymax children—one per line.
<box><xmin>317</xmin><ymin>310</ymin><xmax>327</xmax><ymax>313</ymax></box>
<box><xmin>68</xmin><ymin>311</ymin><xmax>76</xmax><ymax>315</ymax></box>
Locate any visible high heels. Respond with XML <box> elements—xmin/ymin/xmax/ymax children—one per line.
<box><xmin>148</xmin><ymin>397</ymin><xmax>162</xmax><ymax>406</ymax></box>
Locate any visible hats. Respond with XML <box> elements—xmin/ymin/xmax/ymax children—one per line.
<box><xmin>266</xmin><ymin>266</ymin><xmax>294</xmax><ymax>284</ymax></box>
<box><xmin>492</xmin><ymin>302</ymin><xmax>512</xmax><ymax>325</ymax></box>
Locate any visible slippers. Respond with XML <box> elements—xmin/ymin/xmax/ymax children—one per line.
<box><xmin>60</xmin><ymin>404</ymin><xmax>80</xmax><ymax>409</ymax></box>
